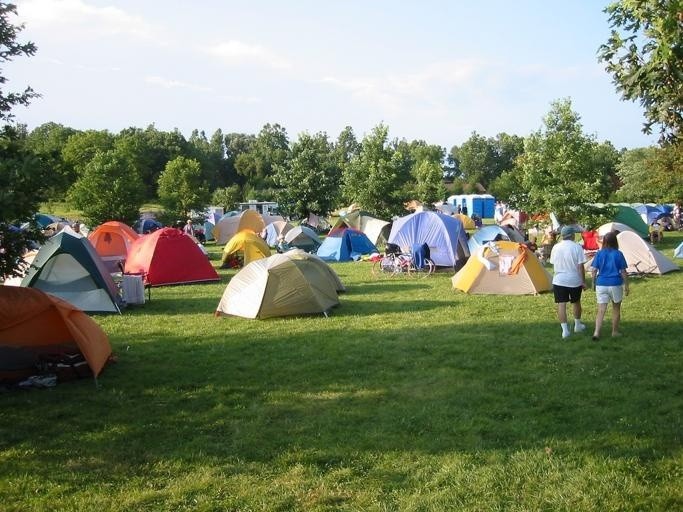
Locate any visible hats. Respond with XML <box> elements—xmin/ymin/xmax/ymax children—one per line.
<box><xmin>560</xmin><ymin>226</ymin><xmax>577</xmax><ymax>238</ymax></box>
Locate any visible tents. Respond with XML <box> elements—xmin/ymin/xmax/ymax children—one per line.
<box><xmin>0</xmin><ymin>201</ymin><xmax>683</xmax><ymax>391</ymax></box>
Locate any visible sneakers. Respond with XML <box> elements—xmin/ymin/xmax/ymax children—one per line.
<box><xmin>574</xmin><ymin>324</ymin><xmax>586</xmax><ymax>333</ymax></box>
<box><xmin>561</xmin><ymin>332</ymin><xmax>571</xmax><ymax>340</ymax></box>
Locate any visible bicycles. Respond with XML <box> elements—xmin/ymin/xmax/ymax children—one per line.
<box><xmin>379</xmin><ymin>250</ymin><xmax>435</xmax><ymax>276</ymax></box>
<box><xmin>369</xmin><ymin>245</ymin><xmax>433</xmax><ymax>281</ymax></box>
